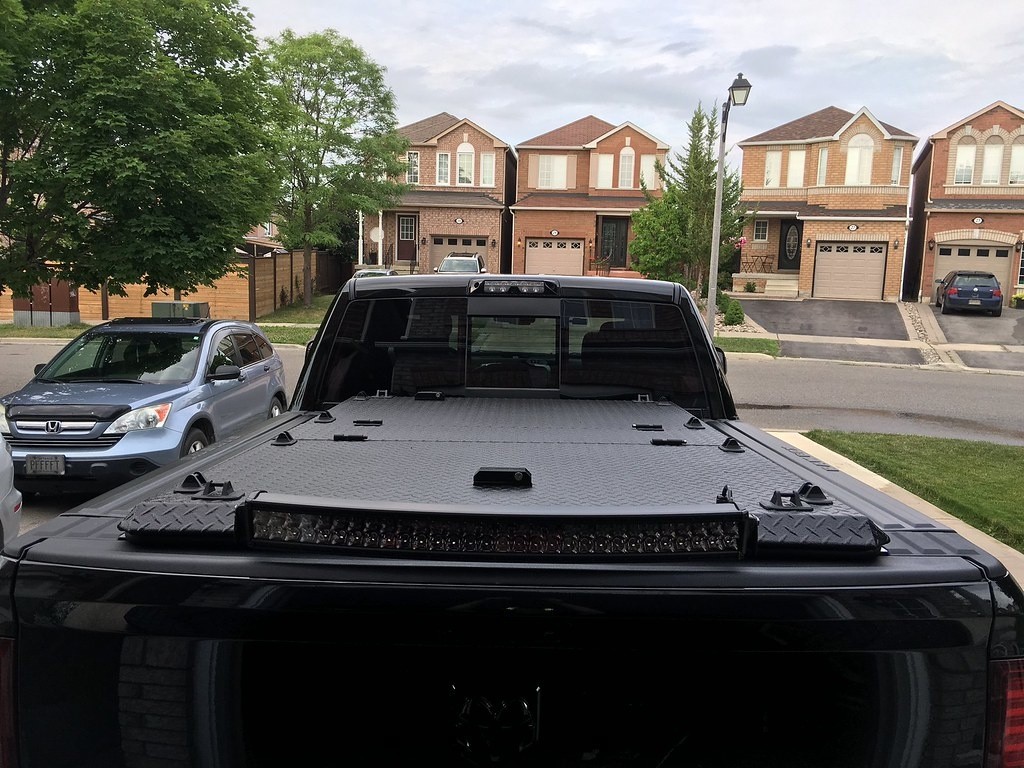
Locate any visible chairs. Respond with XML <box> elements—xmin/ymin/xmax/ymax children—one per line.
<box><xmin>759</xmin><ymin>253</ymin><xmax>775</xmax><ymax>273</ymax></box>
<box><xmin>582</xmin><ymin>321</ymin><xmax>680</xmax><ymax>388</ymax></box>
<box><xmin>390</xmin><ymin>312</ymin><xmax>458</xmax><ymax>395</ymax></box>
<box><xmin>741</xmin><ymin>252</ymin><xmax>754</xmax><ymax>273</ymax></box>
<box><xmin>107</xmin><ymin>344</ymin><xmax>145</xmax><ymax>377</ymax></box>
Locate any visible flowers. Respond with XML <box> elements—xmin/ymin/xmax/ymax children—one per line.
<box><xmin>1010</xmin><ymin>290</ymin><xmax>1024</xmax><ymax>298</ymax></box>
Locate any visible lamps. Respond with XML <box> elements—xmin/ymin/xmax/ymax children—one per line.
<box><xmin>806</xmin><ymin>236</ymin><xmax>811</xmax><ymax>248</ymax></box>
<box><xmin>517</xmin><ymin>237</ymin><xmax>521</xmax><ymax>247</ymax></box>
<box><xmin>422</xmin><ymin>237</ymin><xmax>426</xmax><ymax>245</ymax></box>
<box><xmin>928</xmin><ymin>238</ymin><xmax>935</xmax><ymax>250</ymax></box>
<box><xmin>492</xmin><ymin>239</ymin><xmax>496</xmax><ymax>248</ymax></box>
<box><xmin>894</xmin><ymin>239</ymin><xmax>900</xmax><ymax>250</ymax></box>
<box><xmin>1016</xmin><ymin>240</ymin><xmax>1023</xmax><ymax>252</ymax></box>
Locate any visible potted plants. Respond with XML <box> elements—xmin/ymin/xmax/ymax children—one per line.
<box><xmin>1008</xmin><ymin>298</ymin><xmax>1024</xmax><ymax>309</ymax></box>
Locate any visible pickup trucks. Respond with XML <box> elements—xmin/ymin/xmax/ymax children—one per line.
<box><xmin>1</xmin><ymin>268</ymin><xmax>1024</xmax><ymax>767</ymax></box>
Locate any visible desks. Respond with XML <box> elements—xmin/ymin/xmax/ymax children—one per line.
<box><xmin>750</xmin><ymin>254</ymin><xmax>768</xmax><ymax>274</ymax></box>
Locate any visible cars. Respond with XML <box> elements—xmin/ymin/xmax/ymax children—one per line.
<box><xmin>351</xmin><ymin>268</ymin><xmax>398</xmax><ymax>279</ymax></box>
<box><xmin>935</xmin><ymin>269</ymin><xmax>1003</xmax><ymax>318</ymax></box>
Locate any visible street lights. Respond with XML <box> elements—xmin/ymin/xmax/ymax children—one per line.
<box><xmin>707</xmin><ymin>72</ymin><xmax>754</xmax><ymax>344</ymax></box>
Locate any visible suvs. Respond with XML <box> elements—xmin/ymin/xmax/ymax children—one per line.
<box><xmin>434</xmin><ymin>251</ymin><xmax>487</xmax><ymax>275</ymax></box>
<box><xmin>0</xmin><ymin>316</ymin><xmax>290</xmax><ymax>502</ymax></box>
